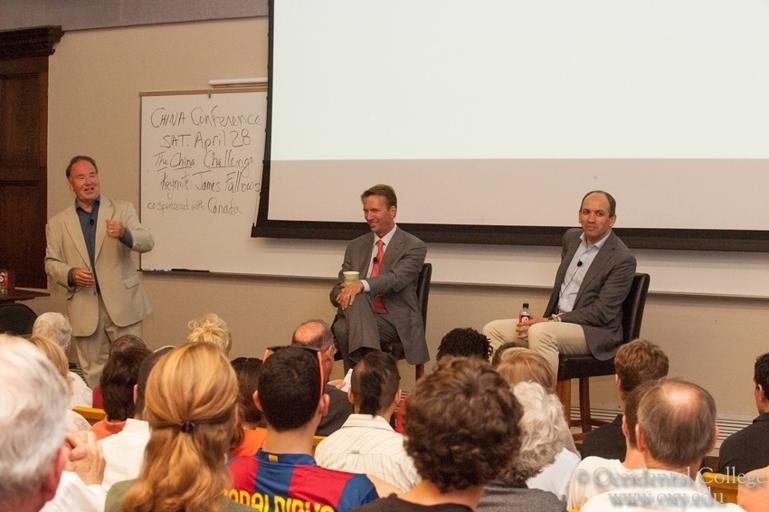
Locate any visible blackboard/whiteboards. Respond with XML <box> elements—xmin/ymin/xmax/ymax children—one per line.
<box><xmin>138</xmin><ymin>87</ymin><xmax>769</xmax><ymax>301</ymax></box>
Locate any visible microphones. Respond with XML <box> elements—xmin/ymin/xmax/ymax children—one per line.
<box><xmin>90</xmin><ymin>219</ymin><xmax>94</xmax><ymax>225</ymax></box>
<box><xmin>374</xmin><ymin>257</ymin><xmax>377</xmax><ymax>263</ymax></box>
<box><xmin>577</xmin><ymin>261</ymin><xmax>582</xmax><ymax>266</ymax></box>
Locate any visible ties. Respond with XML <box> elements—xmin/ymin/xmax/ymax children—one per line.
<box><xmin>369</xmin><ymin>240</ymin><xmax>388</xmax><ymax>315</ymax></box>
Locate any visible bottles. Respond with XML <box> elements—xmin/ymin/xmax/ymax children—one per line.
<box><xmin>518</xmin><ymin>302</ymin><xmax>532</xmax><ymax>337</ymax></box>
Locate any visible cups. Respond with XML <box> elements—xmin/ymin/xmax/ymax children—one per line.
<box><xmin>0</xmin><ymin>270</ymin><xmax>17</xmax><ymax>294</ymax></box>
<box><xmin>342</xmin><ymin>270</ymin><xmax>360</xmax><ymax>283</ymax></box>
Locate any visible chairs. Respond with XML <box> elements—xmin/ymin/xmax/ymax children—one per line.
<box><xmin>556</xmin><ymin>273</ymin><xmax>651</xmax><ymax>442</ymax></box>
<box><xmin>381</xmin><ymin>264</ymin><xmax>432</xmax><ymax>384</ymax></box>
<box><xmin>254</xmin><ymin>423</ymin><xmax>329</xmax><ymax>455</ymax></box>
<box><xmin>69</xmin><ymin>401</ymin><xmax>104</xmax><ymax>431</ymax></box>
<box><xmin>703</xmin><ymin>472</ymin><xmax>744</xmax><ymax>506</ymax></box>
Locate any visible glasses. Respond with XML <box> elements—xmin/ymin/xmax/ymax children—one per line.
<box><xmin>263</xmin><ymin>345</ymin><xmax>324</xmax><ymax>399</ymax></box>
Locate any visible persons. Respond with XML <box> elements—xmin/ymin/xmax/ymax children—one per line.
<box><xmin>0</xmin><ymin>302</ymin><xmax>769</xmax><ymax>512</ymax></box>
<box><xmin>330</xmin><ymin>184</ymin><xmax>429</xmax><ymax>377</ymax></box>
<box><xmin>44</xmin><ymin>155</ymin><xmax>156</xmax><ymax>387</ymax></box>
<box><xmin>484</xmin><ymin>191</ymin><xmax>637</xmax><ymax>389</ymax></box>
